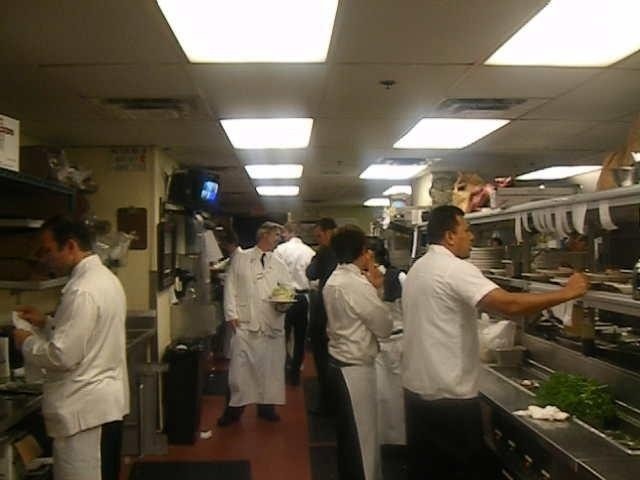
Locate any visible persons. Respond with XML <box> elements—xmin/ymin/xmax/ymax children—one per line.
<box><xmin>305</xmin><ymin>218</ymin><xmax>339</xmax><ymax>416</ymax></box>
<box><xmin>217</xmin><ymin>221</ymin><xmax>295</xmax><ymax>427</ymax></box>
<box><xmin>15</xmin><ymin>215</ymin><xmax>130</xmax><ymax>479</ymax></box>
<box><xmin>321</xmin><ymin>223</ymin><xmax>394</xmax><ymax>480</ymax></box>
<box><xmin>272</xmin><ymin>222</ymin><xmax>320</xmax><ymax>381</ymax></box>
<box><xmin>368</xmin><ymin>236</ymin><xmax>407</xmax><ymax>313</ymax></box>
<box><xmin>400</xmin><ymin>205</ymin><xmax>589</xmax><ymax>480</ymax></box>
<box><xmin>209</xmin><ymin>230</ymin><xmax>244</xmax><ymax>280</ymax></box>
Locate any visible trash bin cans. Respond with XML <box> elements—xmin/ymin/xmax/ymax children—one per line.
<box><xmin>161</xmin><ymin>350</ymin><xmax>201</xmax><ymax>445</ymax></box>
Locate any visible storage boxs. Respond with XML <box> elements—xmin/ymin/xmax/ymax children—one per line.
<box><xmin>0</xmin><ymin>112</ymin><xmax>25</xmax><ymax>174</ymax></box>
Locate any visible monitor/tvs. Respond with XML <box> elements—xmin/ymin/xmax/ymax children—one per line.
<box><xmin>168</xmin><ymin>170</ymin><xmax>224</xmax><ymax>210</ymax></box>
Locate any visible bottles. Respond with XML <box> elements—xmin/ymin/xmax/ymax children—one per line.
<box><xmin>580</xmin><ymin>308</ymin><xmax>595</xmax><ymax>355</ymax></box>
<box><xmin>0</xmin><ymin>337</ymin><xmax>11</xmax><ymax>383</ymax></box>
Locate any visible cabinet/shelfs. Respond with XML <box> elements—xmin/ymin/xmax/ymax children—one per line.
<box><xmin>0</xmin><ymin>162</ymin><xmax>83</xmax><ymax>300</ymax></box>
<box><xmin>410</xmin><ymin>176</ymin><xmax>639</xmax><ymax>425</ymax></box>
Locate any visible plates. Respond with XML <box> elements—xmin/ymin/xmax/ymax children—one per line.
<box><xmin>468</xmin><ymin>248</ymin><xmax>504</xmax><ymax>271</ymax></box>
<box><xmin>261</xmin><ymin>298</ymin><xmax>299</xmax><ymax>304</ymax></box>
<box><xmin>520</xmin><ymin>267</ymin><xmax>633</xmax><ymax>295</ymax></box>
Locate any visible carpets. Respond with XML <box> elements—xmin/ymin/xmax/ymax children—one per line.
<box><xmin>123</xmin><ymin>453</ymin><xmax>257</xmax><ymax>478</ymax></box>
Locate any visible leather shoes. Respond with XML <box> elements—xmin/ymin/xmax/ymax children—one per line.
<box><xmin>258</xmin><ymin>408</ymin><xmax>279</xmax><ymax>421</ymax></box>
<box><xmin>217</xmin><ymin>411</ymin><xmax>239</xmax><ymax>426</ymax></box>
